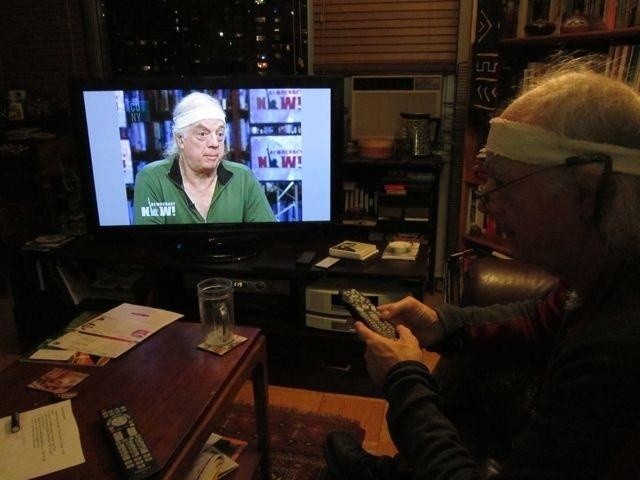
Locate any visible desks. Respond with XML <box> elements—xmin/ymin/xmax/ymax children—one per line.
<box><xmin>0</xmin><ymin>316</ymin><xmax>270</xmax><ymax>480</ymax></box>
<box><xmin>164</xmin><ymin>239</ymin><xmax>432</xmax><ymax>395</ymax></box>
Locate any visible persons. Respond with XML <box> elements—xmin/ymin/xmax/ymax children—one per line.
<box><xmin>319</xmin><ymin>71</ymin><xmax>640</xmax><ymax>479</ymax></box>
<box><xmin>130</xmin><ymin>90</ymin><xmax>282</xmax><ymax>226</ymax></box>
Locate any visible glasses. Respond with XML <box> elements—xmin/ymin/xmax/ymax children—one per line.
<box><xmin>474</xmin><ymin>168</ymin><xmax>547</xmax><ymax>200</ymax></box>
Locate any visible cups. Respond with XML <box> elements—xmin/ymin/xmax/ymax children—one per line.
<box><xmin>389</xmin><ymin>242</ymin><xmax>411</xmax><ymax>254</ymax></box>
<box><xmin>198</xmin><ymin>278</ymin><xmax>236</xmax><ymax>348</ymax></box>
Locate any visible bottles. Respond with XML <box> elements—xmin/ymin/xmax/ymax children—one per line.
<box><xmin>559</xmin><ymin>10</ymin><xmax>591</xmax><ymax>32</ymax></box>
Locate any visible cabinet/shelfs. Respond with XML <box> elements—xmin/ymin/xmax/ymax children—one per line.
<box><xmin>458</xmin><ymin>23</ymin><xmax>639</xmax><ymax>268</ymax></box>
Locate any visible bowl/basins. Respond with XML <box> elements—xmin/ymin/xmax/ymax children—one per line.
<box><xmin>358</xmin><ymin>136</ymin><xmax>395</xmax><ymax>160</ymax></box>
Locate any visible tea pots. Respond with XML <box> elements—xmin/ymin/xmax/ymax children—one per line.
<box><xmin>400</xmin><ymin>110</ymin><xmax>442</xmax><ymax>159</ymax></box>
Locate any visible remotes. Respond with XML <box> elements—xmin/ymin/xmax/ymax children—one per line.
<box><xmin>339</xmin><ymin>287</ymin><xmax>400</xmax><ymax>342</ymax></box>
<box><xmin>99</xmin><ymin>400</ymin><xmax>165</xmax><ymax>480</ymax></box>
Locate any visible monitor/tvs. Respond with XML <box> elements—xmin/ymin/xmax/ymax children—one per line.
<box><xmin>69</xmin><ymin>75</ymin><xmax>344</xmax><ymax>263</ymax></box>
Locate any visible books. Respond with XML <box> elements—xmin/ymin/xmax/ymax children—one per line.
<box><xmin>439</xmin><ymin>247</ymin><xmax>481</xmax><ymax>310</ymax></box>
<box><xmin>514</xmin><ymin>0</ymin><xmax>640</xmax><ymax>98</ymax></box>
<box><xmin>465</xmin><ymin>185</ymin><xmax>508</xmax><ymax>241</ymax></box>
<box><xmin>342</xmin><ymin>169</ymin><xmax>440</xmax><ymax>227</ymax></box>
<box><xmin>56</xmin><ymin>263</ymin><xmax>90</xmax><ymax>306</ymax></box>
<box><xmin>327</xmin><ymin>238</ymin><xmax>376</xmax><ymax>260</ymax></box>
<box><xmin>383</xmin><ymin>240</ymin><xmax>421</xmax><ymax>262</ymax></box>
